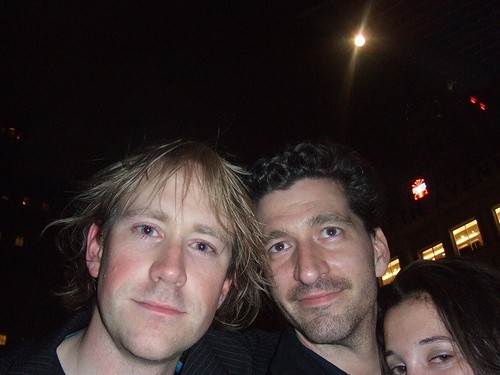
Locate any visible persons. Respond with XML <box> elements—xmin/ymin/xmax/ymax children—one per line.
<box><xmin>376</xmin><ymin>261</ymin><xmax>499</xmax><ymax>375</ymax></box>
<box><xmin>4</xmin><ymin>141</ymin><xmax>274</xmax><ymax>375</ymax></box>
<box><xmin>177</xmin><ymin>139</ymin><xmax>391</xmax><ymax>375</ymax></box>
